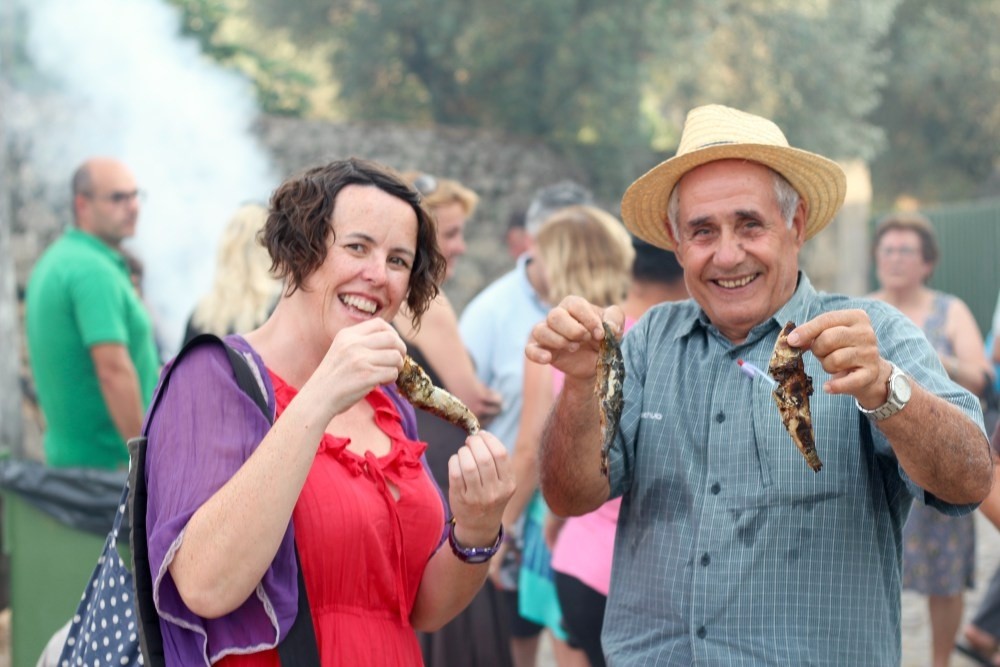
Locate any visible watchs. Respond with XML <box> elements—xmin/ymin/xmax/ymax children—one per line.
<box><xmin>447</xmin><ymin>514</ymin><xmax>505</xmax><ymax>566</ymax></box>
<box><xmin>854</xmin><ymin>358</ymin><xmax>912</xmax><ymax>422</ymax></box>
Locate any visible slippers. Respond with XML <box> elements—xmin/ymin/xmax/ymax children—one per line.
<box><xmin>953</xmin><ymin>642</ymin><xmax>1000</xmax><ymax>667</ymax></box>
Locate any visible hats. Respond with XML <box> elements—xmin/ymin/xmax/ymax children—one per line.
<box><xmin>524</xmin><ymin>180</ymin><xmax>592</xmax><ymax>247</ymax></box>
<box><xmin>620</xmin><ymin>104</ymin><xmax>847</xmax><ymax>251</ymax></box>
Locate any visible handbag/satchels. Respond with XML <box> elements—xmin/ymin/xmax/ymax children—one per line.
<box><xmin>57</xmin><ymin>470</ymin><xmax>145</xmax><ymax>667</ymax></box>
<box><xmin>132</xmin><ymin>333</ymin><xmax>321</xmax><ymax>667</ymax></box>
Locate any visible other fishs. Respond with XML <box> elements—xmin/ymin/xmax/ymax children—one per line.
<box><xmin>392</xmin><ymin>322</ymin><xmax>824</xmax><ymax>475</ymax></box>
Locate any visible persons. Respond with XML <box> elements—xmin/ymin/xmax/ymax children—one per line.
<box><xmin>857</xmin><ymin>214</ymin><xmax>998</xmax><ymax>667</ymax></box>
<box><xmin>144</xmin><ymin>160</ymin><xmax>519</xmax><ymax>667</ymax></box>
<box><xmin>396</xmin><ymin>168</ymin><xmax>693</xmax><ymax>667</ymax></box>
<box><xmin>24</xmin><ymin>155</ymin><xmax>172</xmax><ymax>482</ymax></box>
<box><xmin>523</xmin><ymin>102</ymin><xmax>995</xmax><ymax>667</ymax></box>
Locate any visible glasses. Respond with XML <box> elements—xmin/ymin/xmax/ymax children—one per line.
<box><xmin>92</xmin><ymin>189</ymin><xmax>139</xmax><ymax>202</ymax></box>
<box><xmin>414</xmin><ymin>172</ymin><xmax>437</xmax><ymax>206</ymax></box>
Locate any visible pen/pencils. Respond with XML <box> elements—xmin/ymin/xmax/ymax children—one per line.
<box><xmin>737</xmin><ymin>359</ymin><xmax>775</xmax><ymax>385</ymax></box>
